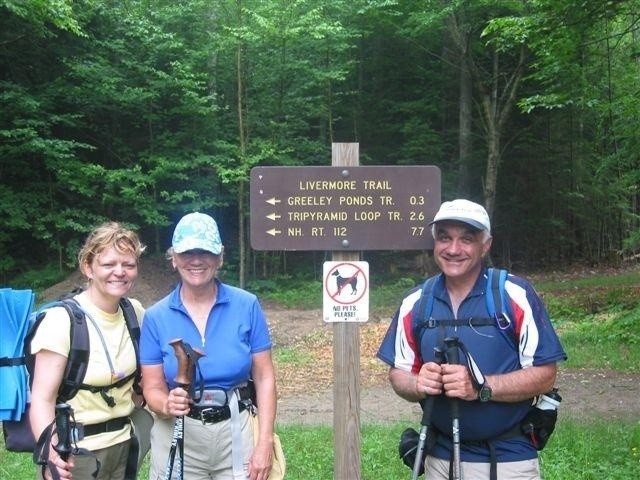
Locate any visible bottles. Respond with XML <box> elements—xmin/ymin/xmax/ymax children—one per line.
<box><xmin>171</xmin><ymin>210</ymin><xmax>223</xmax><ymax>256</ymax></box>
<box><xmin>427</xmin><ymin>197</ymin><xmax>491</xmax><ymax>233</ymax></box>
<box><xmin>1</xmin><ymin>297</ymin><xmax>91</xmax><ymax>453</ymax></box>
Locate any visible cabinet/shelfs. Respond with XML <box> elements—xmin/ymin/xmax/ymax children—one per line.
<box><xmin>535</xmin><ymin>388</ymin><xmax>562</xmax><ymax>410</ymax></box>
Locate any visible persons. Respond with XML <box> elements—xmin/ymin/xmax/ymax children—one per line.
<box><xmin>374</xmin><ymin>199</ymin><xmax>568</xmax><ymax>480</ymax></box>
<box><xmin>28</xmin><ymin>221</ymin><xmax>150</xmax><ymax>480</ymax></box>
<box><xmin>139</xmin><ymin>210</ymin><xmax>277</xmax><ymax>480</ymax></box>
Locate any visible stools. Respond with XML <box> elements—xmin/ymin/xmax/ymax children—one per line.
<box><xmin>183</xmin><ymin>399</ymin><xmax>257</xmax><ymax>426</ymax></box>
